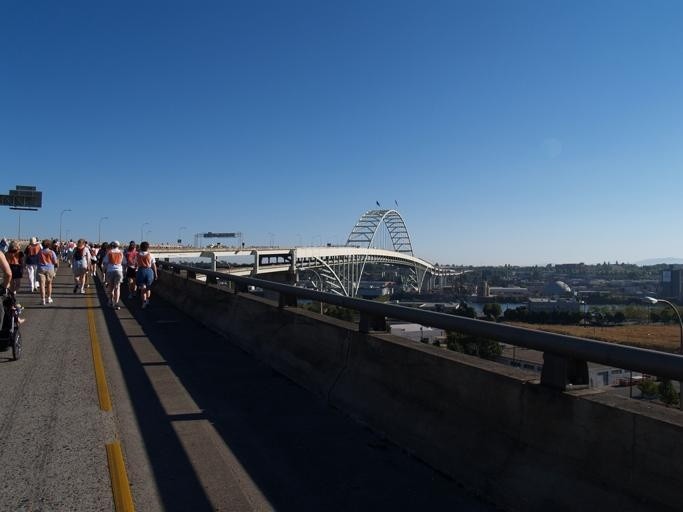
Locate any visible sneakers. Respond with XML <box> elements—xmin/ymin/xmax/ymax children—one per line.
<box><xmin>73</xmin><ymin>283</ymin><xmax>152</xmax><ymax>310</ymax></box>
<box><xmin>47</xmin><ymin>297</ymin><xmax>53</xmax><ymax>303</ymax></box>
<box><xmin>40</xmin><ymin>299</ymin><xmax>46</xmax><ymax>305</ymax></box>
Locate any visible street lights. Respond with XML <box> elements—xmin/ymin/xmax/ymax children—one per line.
<box><xmin>141</xmin><ymin>222</ymin><xmax>149</xmax><ymax>240</ymax></box>
<box><xmin>297</xmin><ymin>267</ymin><xmax>323</xmax><ymax>315</ymax></box>
<box><xmin>640</xmin><ymin>295</ymin><xmax>682</xmax><ymax>355</ymax></box>
<box><xmin>98</xmin><ymin>217</ymin><xmax>107</xmax><ymax>242</ymax></box>
<box><xmin>580</xmin><ymin>300</ymin><xmax>586</xmax><ymax>328</ymax></box>
<box><xmin>59</xmin><ymin>209</ymin><xmax>71</xmax><ymax>242</ymax></box>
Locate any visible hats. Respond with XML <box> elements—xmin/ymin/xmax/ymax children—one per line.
<box><xmin>30</xmin><ymin>237</ymin><xmax>36</xmax><ymax>246</ymax></box>
<box><xmin>112</xmin><ymin>241</ymin><xmax>119</xmax><ymax>247</ymax></box>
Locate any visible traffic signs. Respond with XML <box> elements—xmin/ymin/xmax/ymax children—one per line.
<box><xmin>9</xmin><ymin>190</ymin><xmax>41</xmax><ymax>208</ymax></box>
<box><xmin>203</xmin><ymin>233</ymin><xmax>234</xmax><ymax>238</ymax></box>
<box><xmin>16</xmin><ymin>185</ymin><xmax>35</xmax><ymax>191</ymax></box>
<box><xmin>0</xmin><ymin>195</ymin><xmax>23</xmax><ymax>206</ymax></box>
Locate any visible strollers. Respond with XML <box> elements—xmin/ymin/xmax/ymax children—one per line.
<box><xmin>0</xmin><ymin>286</ymin><xmax>25</xmax><ymax>360</ymax></box>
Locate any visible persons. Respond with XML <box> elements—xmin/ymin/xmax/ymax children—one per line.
<box><xmin>1</xmin><ymin>237</ymin><xmax>158</xmax><ymax>332</ymax></box>
